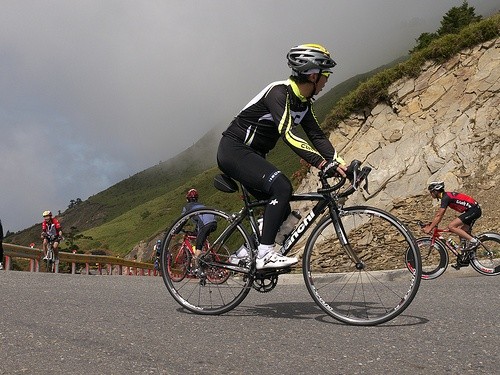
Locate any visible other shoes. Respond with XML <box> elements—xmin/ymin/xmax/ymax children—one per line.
<box><xmin>55</xmin><ymin>259</ymin><xmax>59</xmax><ymax>265</ymax></box>
<box><xmin>43</xmin><ymin>257</ymin><xmax>47</xmax><ymax>261</ymax></box>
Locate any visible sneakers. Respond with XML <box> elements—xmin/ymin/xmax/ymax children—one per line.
<box><xmin>465</xmin><ymin>238</ymin><xmax>481</xmax><ymax>250</ymax></box>
<box><xmin>256</xmin><ymin>243</ymin><xmax>298</xmax><ymax>269</ymax></box>
<box><xmin>228</xmin><ymin>253</ymin><xmax>253</xmax><ymax>266</ymax></box>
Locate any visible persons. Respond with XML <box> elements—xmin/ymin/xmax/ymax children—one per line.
<box><xmin>174</xmin><ymin>188</ymin><xmax>217</xmax><ymax>286</ymax></box>
<box><xmin>151</xmin><ymin>239</ymin><xmax>162</xmax><ymax>270</ymax></box>
<box><xmin>41</xmin><ymin>211</ymin><xmax>62</xmax><ymax>264</ymax></box>
<box><xmin>216</xmin><ymin>43</ymin><xmax>348</xmax><ymax>275</ymax></box>
<box><xmin>0</xmin><ymin>220</ymin><xmax>4</xmax><ymax>270</ymax></box>
<box><xmin>421</xmin><ymin>181</ymin><xmax>482</xmax><ymax>266</ymax></box>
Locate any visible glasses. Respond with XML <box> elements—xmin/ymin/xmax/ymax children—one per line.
<box><xmin>321</xmin><ymin>70</ymin><xmax>331</xmax><ymax>77</ymax></box>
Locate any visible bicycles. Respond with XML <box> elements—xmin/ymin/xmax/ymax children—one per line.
<box><xmin>159</xmin><ymin>158</ymin><xmax>423</xmax><ymax>326</ymax></box>
<box><xmin>167</xmin><ymin>227</ymin><xmax>232</xmax><ymax>285</ymax></box>
<box><xmin>42</xmin><ymin>232</ymin><xmax>62</xmax><ymax>273</ymax></box>
<box><xmin>151</xmin><ymin>256</ymin><xmax>163</xmax><ymax>277</ymax></box>
<box><xmin>404</xmin><ymin>220</ymin><xmax>500</xmax><ymax>280</ymax></box>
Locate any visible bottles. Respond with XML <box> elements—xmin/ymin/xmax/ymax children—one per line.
<box><xmin>191</xmin><ymin>244</ymin><xmax>196</xmax><ymax>254</ymax></box>
<box><xmin>460</xmin><ymin>237</ymin><xmax>466</xmax><ymax>245</ymax></box>
<box><xmin>257</xmin><ymin>216</ymin><xmax>263</xmax><ymax>236</ymax></box>
<box><xmin>202</xmin><ymin>247</ymin><xmax>206</xmax><ymax>252</ymax></box>
<box><xmin>274</xmin><ymin>211</ymin><xmax>302</xmax><ymax>245</ymax></box>
<box><xmin>448</xmin><ymin>238</ymin><xmax>459</xmax><ymax>249</ymax></box>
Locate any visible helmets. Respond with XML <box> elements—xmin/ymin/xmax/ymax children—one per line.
<box><xmin>43</xmin><ymin>210</ymin><xmax>52</xmax><ymax>217</ymax></box>
<box><xmin>428</xmin><ymin>181</ymin><xmax>444</xmax><ymax>191</ymax></box>
<box><xmin>286</xmin><ymin>44</ymin><xmax>337</xmax><ymax>71</ymax></box>
<box><xmin>156</xmin><ymin>240</ymin><xmax>161</xmax><ymax>244</ymax></box>
<box><xmin>186</xmin><ymin>189</ymin><xmax>199</xmax><ymax>198</ymax></box>
